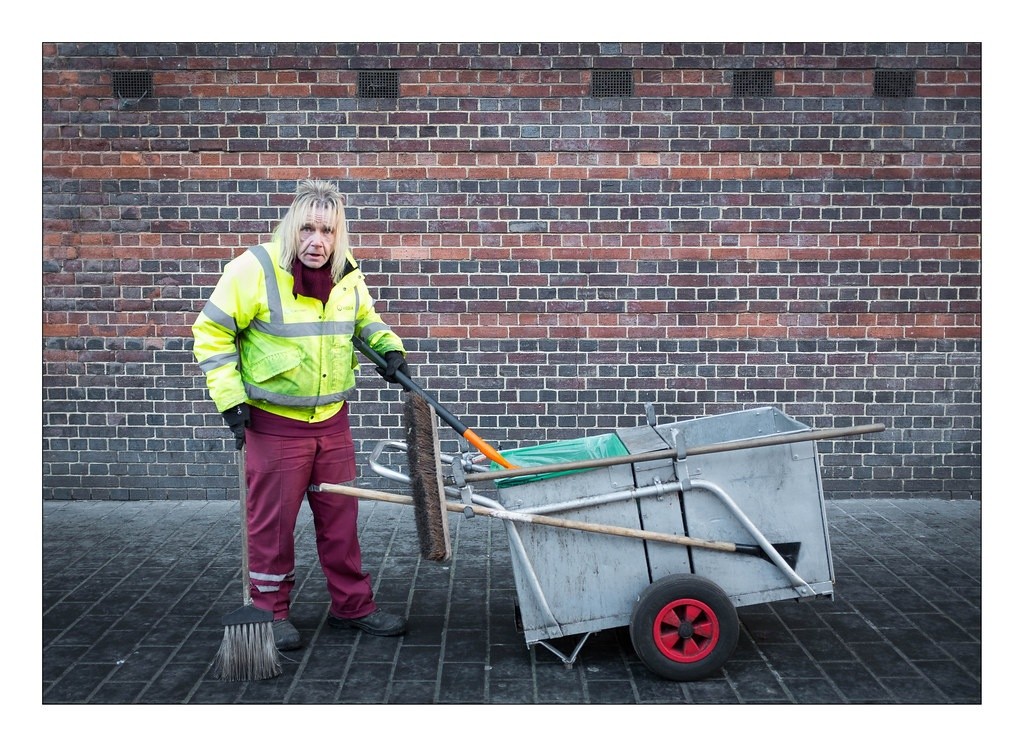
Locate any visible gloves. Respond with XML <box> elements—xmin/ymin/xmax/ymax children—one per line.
<box><xmin>374</xmin><ymin>351</ymin><xmax>414</xmax><ymax>392</ymax></box>
<box><xmin>221</xmin><ymin>402</ymin><xmax>251</xmax><ymax>451</ymax></box>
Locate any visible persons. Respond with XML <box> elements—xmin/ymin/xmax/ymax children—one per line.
<box><xmin>191</xmin><ymin>180</ymin><xmax>411</xmax><ymax>652</ymax></box>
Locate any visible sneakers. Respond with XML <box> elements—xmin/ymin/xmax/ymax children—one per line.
<box><xmin>271</xmin><ymin>619</ymin><xmax>304</xmax><ymax>650</ymax></box>
<box><xmin>328</xmin><ymin>607</ymin><xmax>408</xmax><ymax>636</ymax></box>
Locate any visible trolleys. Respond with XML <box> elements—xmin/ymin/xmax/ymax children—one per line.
<box><xmin>320</xmin><ymin>407</ymin><xmax>837</xmax><ymax>684</ymax></box>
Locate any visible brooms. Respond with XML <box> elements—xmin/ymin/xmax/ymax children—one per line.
<box><xmin>210</xmin><ymin>446</ymin><xmax>286</xmax><ymax>681</ymax></box>
